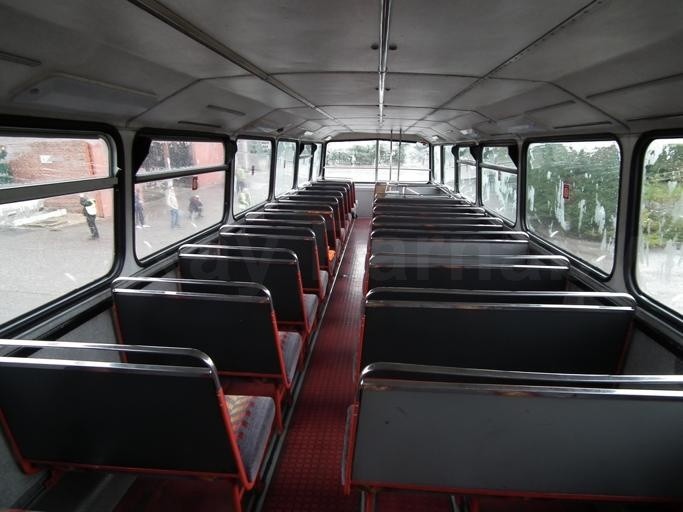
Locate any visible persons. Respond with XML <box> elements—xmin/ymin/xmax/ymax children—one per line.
<box><xmin>80</xmin><ymin>195</ymin><xmax>99</xmax><ymax>240</ymax></box>
<box><xmin>189</xmin><ymin>194</ymin><xmax>204</xmax><ymax>219</ymax></box>
<box><xmin>135</xmin><ymin>187</ymin><xmax>145</xmax><ymax>229</ymax></box>
<box><xmin>166</xmin><ymin>186</ymin><xmax>179</xmax><ymax>228</ymax></box>
<box><xmin>237</xmin><ymin>167</ymin><xmax>246</xmax><ymax>195</ymax></box>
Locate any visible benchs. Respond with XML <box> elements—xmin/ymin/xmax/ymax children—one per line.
<box><xmin>340</xmin><ymin>361</ymin><xmax>682</xmax><ymax>512</ymax></box>
<box><xmin>110</xmin><ymin>277</ymin><xmax>303</xmax><ymax>430</ymax></box>
<box><xmin>1</xmin><ymin>339</ymin><xmax>277</xmax><ymax>510</ymax></box>
<box><xmin>363</xmin><ymin>180</ymin><xmax>570</xmax><ymax>305</ymax></box>
<box><xmin>355</xmin><ymin>287</ymin><xmax>637</xmax><ymax>387</ymax></box>
<box><xmin>177</xmin><ymin>244</ymin><xmax>318</xmax><ymax>351</ymax></box>
<box><xmin>216</xmin><ymin>180</ymin><xmax>358</xmax><ymax>302</ymax></box>
